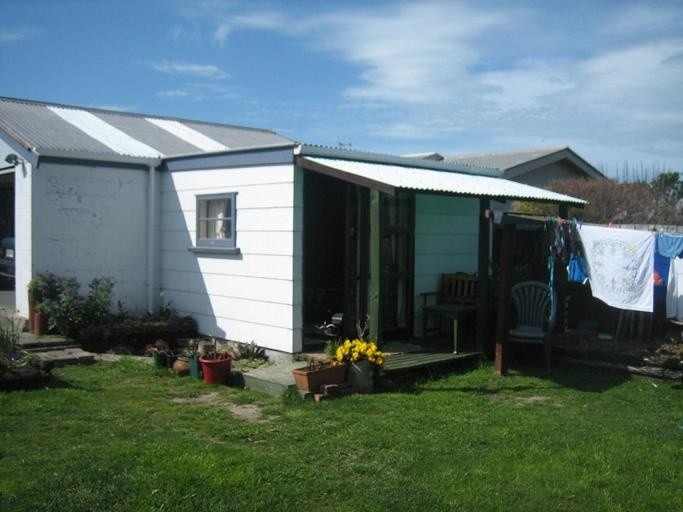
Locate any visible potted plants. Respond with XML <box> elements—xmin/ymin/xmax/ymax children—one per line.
<box><xmin>0</xmin><ymin>268</ymin><xmax>234</xmax><ymax>387</ymax></box>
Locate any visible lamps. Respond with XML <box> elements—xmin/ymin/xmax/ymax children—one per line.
<box><xmin>4</xmin><ymin>152</ymin><xmax>22</xmax><ymax>166</ymax></box>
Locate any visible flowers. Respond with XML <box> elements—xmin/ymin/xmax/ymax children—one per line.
<box><xmin>335</xmin><ymin>336</ymin><xmax>385</xmax><ymax>366</ymax></box>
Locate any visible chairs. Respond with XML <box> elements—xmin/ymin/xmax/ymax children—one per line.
<box><xmin>418</xmin><ymin>270</ymin><xmax>479</xmax><ymax>354</ymax></box>
<box><xmin>508</xmin><ymin>279</ymin><xmax>558</xmax><ymax>374</ymax></box>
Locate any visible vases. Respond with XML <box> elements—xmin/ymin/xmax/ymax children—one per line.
<box><xmin>349</xmin><ymin>360</ymin><xmax>378</xmax><ymax>394</ymax></box>
<box><xmin>290</xmin><ymin>360</ymin><xmax>347</xmax><ymax>391</ymax></box>
